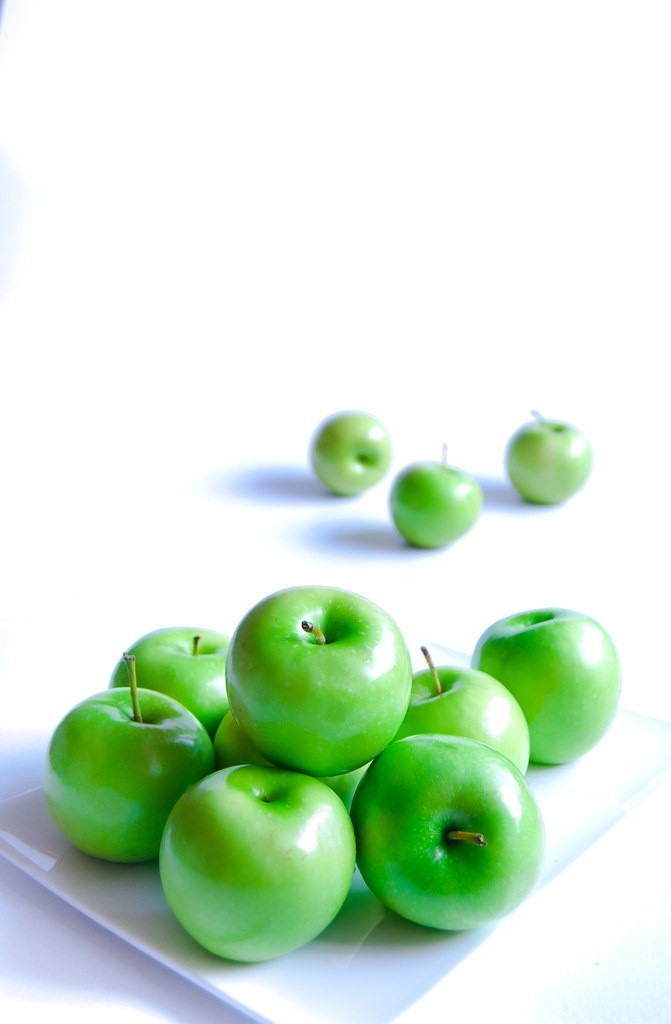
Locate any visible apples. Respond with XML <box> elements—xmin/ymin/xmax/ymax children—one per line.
<box><xmin>308</xmin><ymin>406</ymin><xmax>393</xmax><ymax>498</ymax></box>
<box><xmin>501</xmin><ymin>410</ymin><xmax>593</xmax><ymax>505</ymax></box>
<box><xmin>42</xmin><ymin>585</ymin><xmax>618</xmax><ymax>968</ymax></box>
<box><xmin>389</xmin><ymin>440</ymin><xmax>484</xmax><ymax>550</ymax></box>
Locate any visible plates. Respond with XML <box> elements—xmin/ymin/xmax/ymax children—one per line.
<box><xmin>0</xmin><ymin>644</ymin><xmax>670</xmax><ymax>1024</ymax></box>
